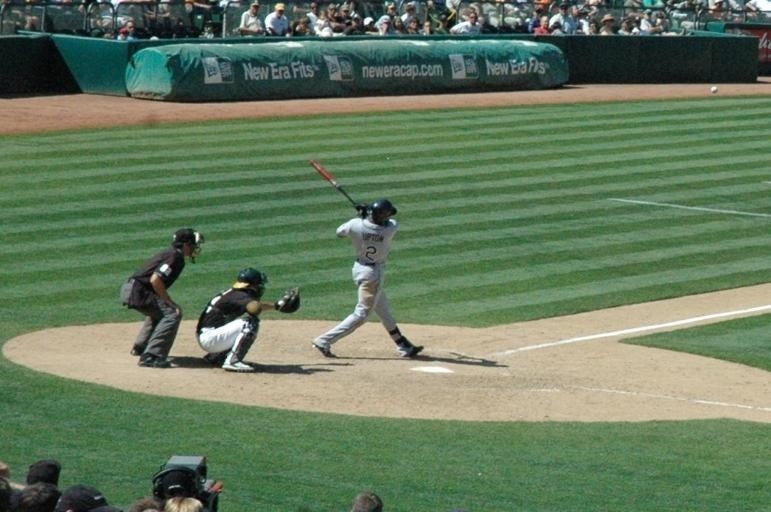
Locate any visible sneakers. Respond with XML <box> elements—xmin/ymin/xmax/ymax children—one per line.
<box><xmin>203</xmin><ymin>353</ymin><xmax>221</xmax><ymax>365</ymax></box>
<box><xmin>400</xmin><ymin>345</ymin><xmax>424</xmax><ymax>358</ymax></box>
<box><xmin>312</xmin><ymin>339</ymin><xmax>337</xmax><ymax>358</ymax></box>
<box><xmin>130</xmin><ymin>343</ymin><xmax>145</xmax><ymax>355</ymax></box>
<box><xmin>221</xmin><ymin>352</ymin><xmax>256</xmax><ymax>373</ymax></box>
<box><xmin>138</xmin><ymin>352</ymin><xmax>170</xmax><ymax>367</ymax></box>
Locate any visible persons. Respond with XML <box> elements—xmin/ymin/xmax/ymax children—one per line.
<box><xmin>120</xmin><ymin>228</ymin><xmax>206</xmax><ymax>368</ymax></box>
<box><xmin>310</xmin><ymin>199</ymin><xmax>425</xmax><ymax>359</ymax></box>
<box><xmin>195</xmin><ymin>266</ymin><xmax>301</xmax><ymax>373</ymax></box>
<box><xmin>0</xmin><ymin>453</ymin><xmax>225</xmax><ymax>512</ymax></box>
<box><xmin>350</xmin><ymin>491</ymin><xmax>383</xmax><ymax>512</ymax></box>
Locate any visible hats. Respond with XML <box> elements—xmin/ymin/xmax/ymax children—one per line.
<box><xmin>27</xmin><ymin>458</ymin><xmax>62</xmax><ymax>485</ymax></box>
<box><xmin>53</xmin><ymin>483</ymin><xmax>105</xmax><ymax>512</ymax></box>
<box><xmin>243</xmin><ymin>2</ymin><xmax>653</xmax><ymax>28</ymax></box>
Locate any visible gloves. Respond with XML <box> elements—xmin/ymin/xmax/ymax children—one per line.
<box><xmin>354</xmin><ymin>201</ymin><xmax>371</xmax><ymax>219</ymax></box>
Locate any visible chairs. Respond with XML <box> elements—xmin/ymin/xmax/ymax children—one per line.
<box><xmin>1</xmin><ymin>0</ymin><xmax>460</xmax><ymax>42</ymax></box>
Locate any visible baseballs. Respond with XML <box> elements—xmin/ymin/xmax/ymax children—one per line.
<box><xmin>712</xmin><ymin>87</ymin><xmax>718</xmax><ymax>93</ymax></box>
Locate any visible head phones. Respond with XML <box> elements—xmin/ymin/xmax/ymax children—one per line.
<box><xmin>151</xmin><ymin>466</ymin><xmax>202</xmax><ymax>498</ymax></box>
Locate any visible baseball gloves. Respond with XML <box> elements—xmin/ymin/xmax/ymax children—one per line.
<box><xmin>274</xmin><ymin>287</ymin><xmax>300</xmax><ymax>312</ymax></box>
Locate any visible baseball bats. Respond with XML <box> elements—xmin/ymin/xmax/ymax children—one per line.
<box><xmin>311</xmin><ymin>160</ymin><xmax>365</xmax><ymax>211</ymax></box>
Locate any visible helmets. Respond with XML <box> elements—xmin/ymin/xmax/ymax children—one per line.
<box><xmin>238</xmin><ymin>268</ymin><xmax>268</xmax><ymax>297</ymax></box>
<box><xmin>172</xmin><ymin>228</ymin><xmax>205</xmax><ymax>258</ymax></box>
<box><xmin>371</xmin><ymin>200</ymin><xmax>396</xmax><ymax>217</ymax></box>
